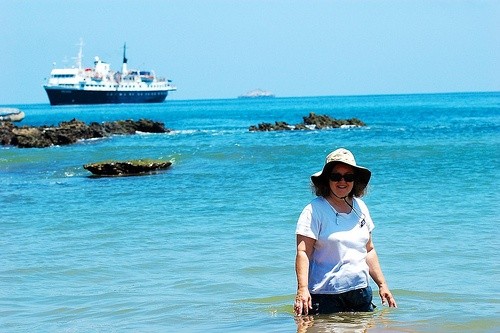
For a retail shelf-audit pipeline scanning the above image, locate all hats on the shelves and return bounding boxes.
[306,145,372,197]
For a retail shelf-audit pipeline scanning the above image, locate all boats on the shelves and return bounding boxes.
[43,37,177,106]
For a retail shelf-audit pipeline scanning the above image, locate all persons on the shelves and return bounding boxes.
[294,148,398,316]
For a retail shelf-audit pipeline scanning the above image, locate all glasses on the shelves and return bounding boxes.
[327,171,360,185]
